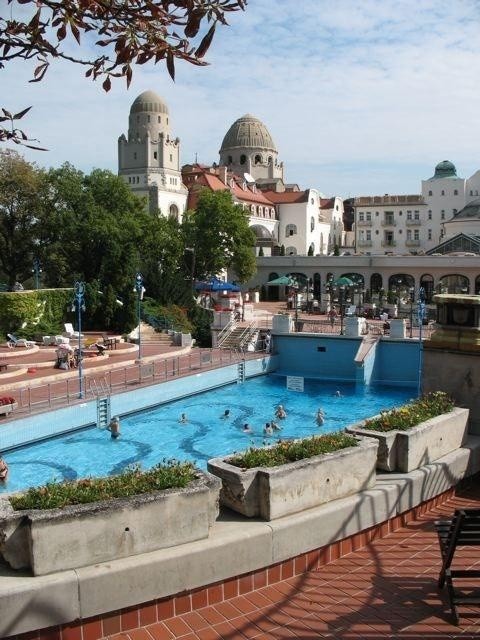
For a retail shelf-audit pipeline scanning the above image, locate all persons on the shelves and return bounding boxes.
[333,390,342,398]
[327,305,338,328]
[220,404,325,447]
[106,415,121,440]
[178,413,188,424]
[0,455,8,484]
[264,330,272,354]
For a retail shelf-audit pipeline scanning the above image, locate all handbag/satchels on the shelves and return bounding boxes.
[59,363,68,370]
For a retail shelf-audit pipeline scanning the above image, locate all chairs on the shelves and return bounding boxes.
[301,301,382,320]
[0,323,118,372]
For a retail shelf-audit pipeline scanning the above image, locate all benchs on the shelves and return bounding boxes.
[432,507,480,627]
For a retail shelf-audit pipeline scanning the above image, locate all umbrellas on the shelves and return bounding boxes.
[265,275,298,300]
[194,276,239,293]
[325,277,357,289]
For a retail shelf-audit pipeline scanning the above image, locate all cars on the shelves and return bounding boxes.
[0,281,26,291]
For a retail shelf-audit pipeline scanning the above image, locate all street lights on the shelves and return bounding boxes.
[69,280,87,400]
[30,257,44,290]
[286,273,416,339]
[132,271,147,364]
[184,247,195,291]
